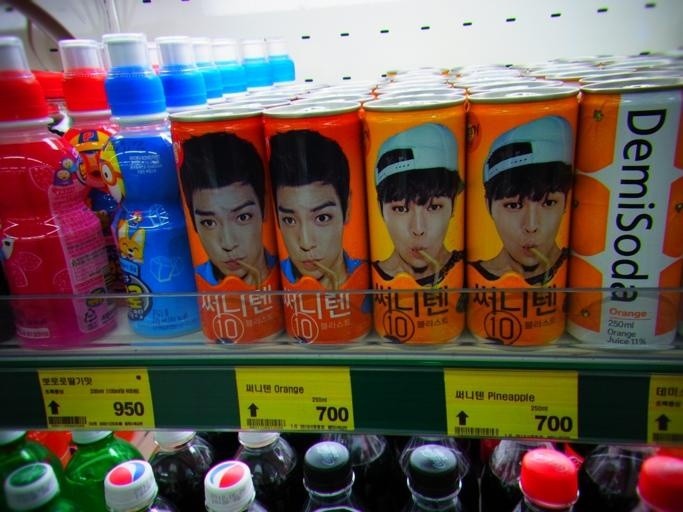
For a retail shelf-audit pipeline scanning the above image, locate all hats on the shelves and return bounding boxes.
[375,124,466,195]
[482,115,575,186]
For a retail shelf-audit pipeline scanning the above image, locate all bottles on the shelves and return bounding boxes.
[0,426,682,511]
[0,31,298,347]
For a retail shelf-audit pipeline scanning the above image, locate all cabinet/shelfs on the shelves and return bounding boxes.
[0,0,683,512]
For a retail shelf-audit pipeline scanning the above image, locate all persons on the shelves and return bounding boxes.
[179,132,278,285]
[370,122,464,285]
[267,129,362,284]
[466,117,574,285]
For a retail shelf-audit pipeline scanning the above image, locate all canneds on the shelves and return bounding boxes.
[168,50,682,349]
